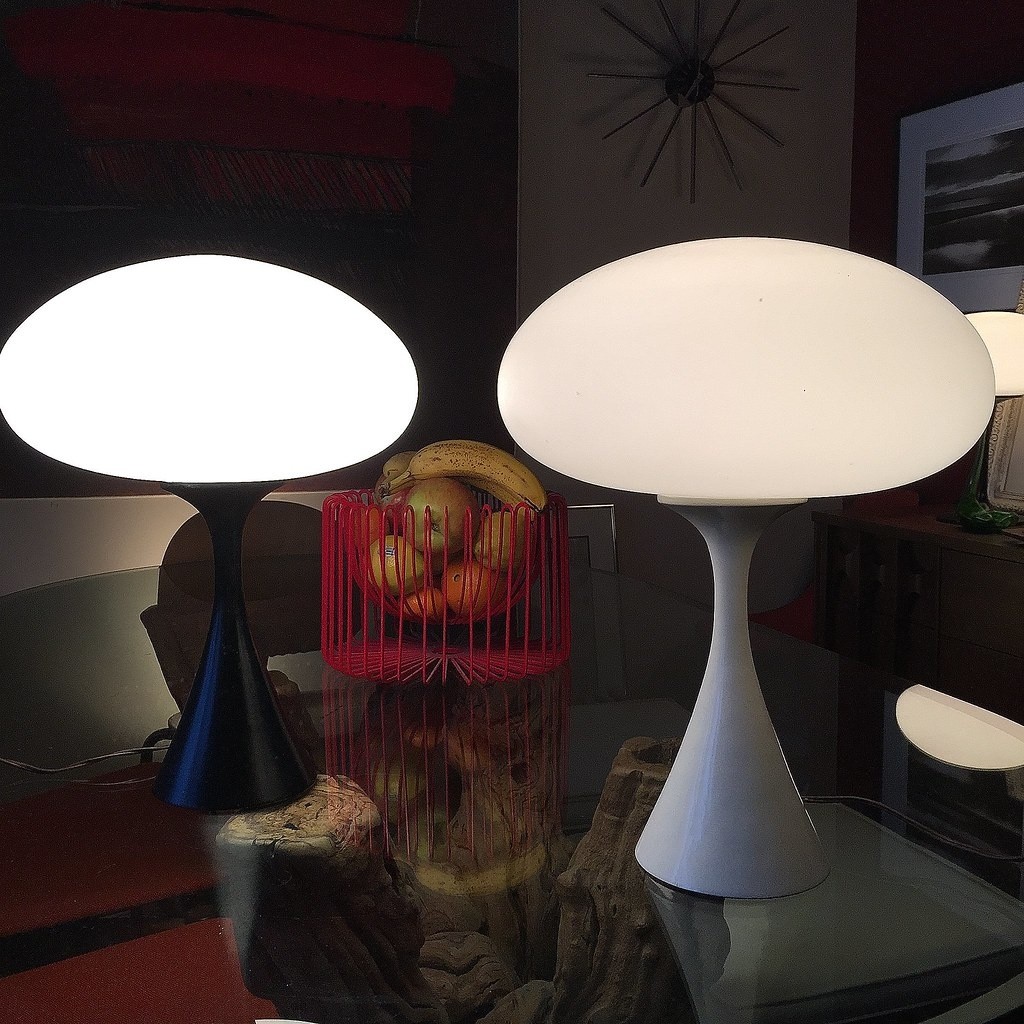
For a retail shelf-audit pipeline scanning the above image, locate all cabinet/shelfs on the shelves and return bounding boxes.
[811,506,1024,724]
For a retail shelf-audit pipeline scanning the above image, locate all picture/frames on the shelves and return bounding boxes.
[897,82,1024,310]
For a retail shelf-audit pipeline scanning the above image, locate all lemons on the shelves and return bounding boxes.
[361,534,427,596]
[471,511,536,572]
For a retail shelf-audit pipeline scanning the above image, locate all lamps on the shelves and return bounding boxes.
[936,310,1024,533]
[0,254,419,819]
[495,236,997,901]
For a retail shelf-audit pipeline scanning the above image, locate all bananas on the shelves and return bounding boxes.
[378,440,547,516]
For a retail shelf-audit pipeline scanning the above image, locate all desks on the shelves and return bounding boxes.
[0,555,1024,1024]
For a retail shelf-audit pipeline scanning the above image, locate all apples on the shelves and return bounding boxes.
[401,478,480,554]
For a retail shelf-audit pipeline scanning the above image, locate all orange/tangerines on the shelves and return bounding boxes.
[346,505,510,621]
